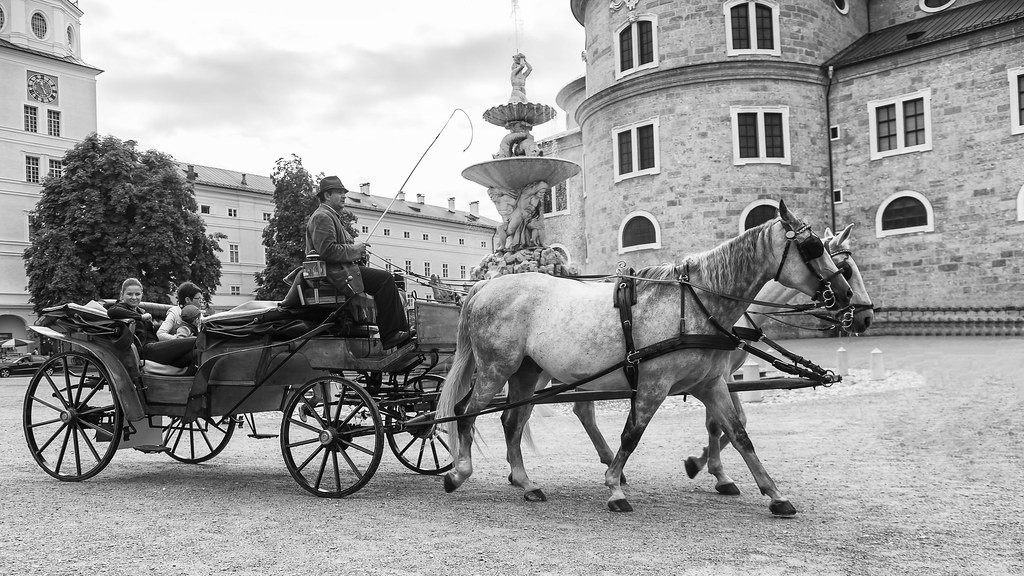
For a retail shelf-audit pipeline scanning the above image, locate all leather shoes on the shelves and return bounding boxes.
[397,329,417,348]
[382,330,412,350]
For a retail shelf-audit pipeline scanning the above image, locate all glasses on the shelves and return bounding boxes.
[189,297,203,302]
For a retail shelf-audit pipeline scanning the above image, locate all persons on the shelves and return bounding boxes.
[22,358,29,363]
[106,278,160,345]
[155,283,205,342]
[32,347,39,355]
[510,52,533,87]
[304,174,416,350]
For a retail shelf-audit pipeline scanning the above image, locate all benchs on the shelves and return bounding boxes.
[96,299,177,336]
[298,266,375,322]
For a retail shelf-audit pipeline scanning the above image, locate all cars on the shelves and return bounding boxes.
[0,355,64,378]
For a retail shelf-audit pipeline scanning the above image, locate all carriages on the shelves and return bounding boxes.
[23,200,876,520]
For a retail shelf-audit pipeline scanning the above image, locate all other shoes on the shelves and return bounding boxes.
[299,407,307,422]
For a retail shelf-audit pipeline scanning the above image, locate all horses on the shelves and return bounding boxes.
[433,198,875,519]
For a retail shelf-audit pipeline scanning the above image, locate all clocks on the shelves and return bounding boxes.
[26,73,58,104]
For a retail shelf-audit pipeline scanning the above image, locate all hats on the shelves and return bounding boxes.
[179,305,201,321]
[317,175,349,195]
[176,281,202,305]
